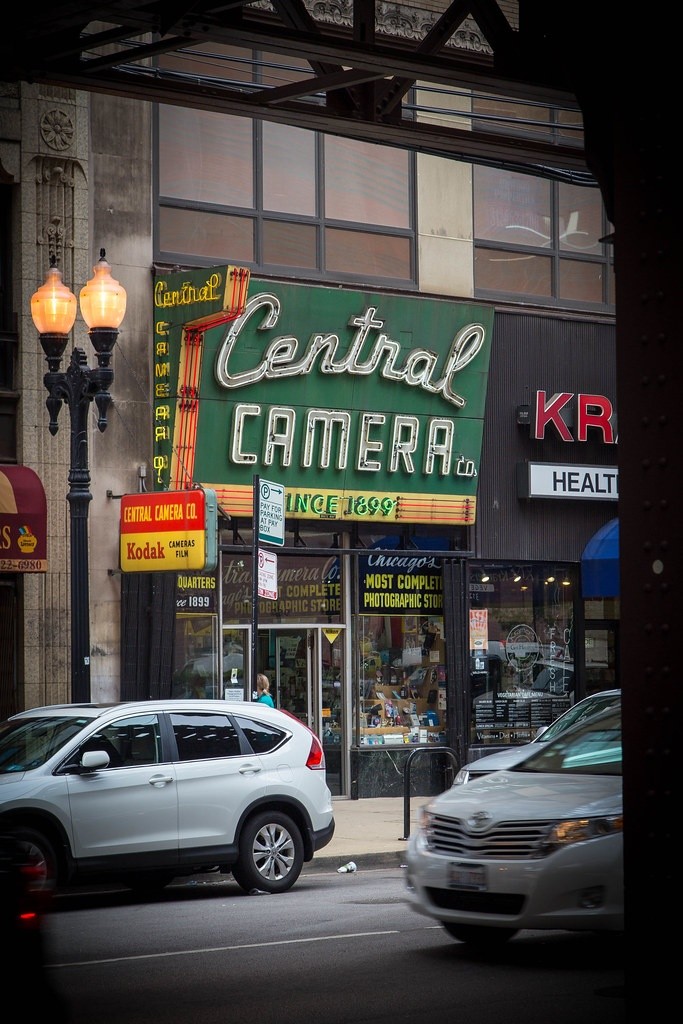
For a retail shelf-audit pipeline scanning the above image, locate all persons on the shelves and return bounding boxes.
[257,673,275,708]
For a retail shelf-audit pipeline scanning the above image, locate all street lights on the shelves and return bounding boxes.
[32,245,128,710]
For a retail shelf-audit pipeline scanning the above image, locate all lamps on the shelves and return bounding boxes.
[480,568,489,582]
[511,568,521,582]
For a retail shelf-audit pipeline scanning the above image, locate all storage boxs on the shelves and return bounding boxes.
[363,713,441,746]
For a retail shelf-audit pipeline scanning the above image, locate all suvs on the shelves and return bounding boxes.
[448,688,622,792]
[406,703,624,946]
[0,696,338,915]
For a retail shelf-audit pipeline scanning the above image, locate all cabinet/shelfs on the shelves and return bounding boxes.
[325,635,444,748]
